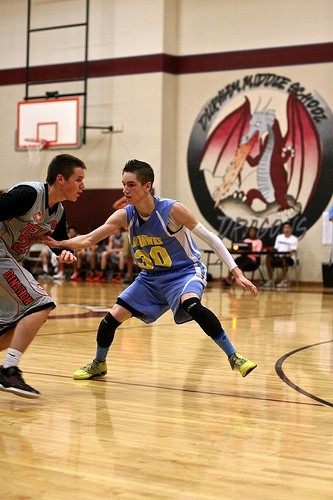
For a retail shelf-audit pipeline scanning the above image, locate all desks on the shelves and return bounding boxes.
[204,249,291,292]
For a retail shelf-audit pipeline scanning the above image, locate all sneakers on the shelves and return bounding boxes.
[227,351,258,377]
[0,365,40,398]
[73,359,107,380]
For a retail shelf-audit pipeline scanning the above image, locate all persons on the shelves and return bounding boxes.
[0,154,86,398]
[41,159,258,379]
[264,222,299,286]
[38,226,134,284]
[224,227,263,286]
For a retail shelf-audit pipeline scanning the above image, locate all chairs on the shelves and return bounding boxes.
[233,255,300,288]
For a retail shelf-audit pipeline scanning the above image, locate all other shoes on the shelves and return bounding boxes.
[221,278,233,286]
[37,270,134,283]
[264,280,272,286]
[276,280,291,287]
